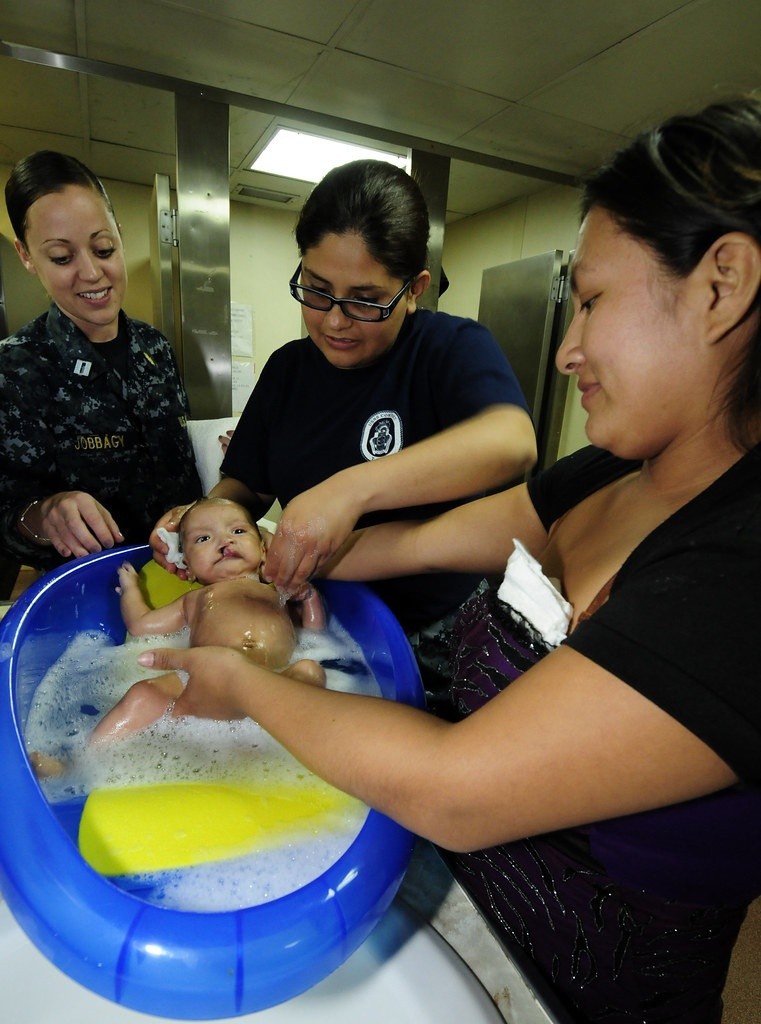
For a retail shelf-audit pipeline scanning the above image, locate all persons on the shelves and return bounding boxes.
[29,497,326,781]
[138,92,761,1024]
[149,159,537,639]
[0,150,234,566]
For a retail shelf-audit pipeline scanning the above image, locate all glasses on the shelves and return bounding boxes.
[289,259,432,322]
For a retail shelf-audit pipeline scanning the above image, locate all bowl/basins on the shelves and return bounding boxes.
[0,545,426,1021]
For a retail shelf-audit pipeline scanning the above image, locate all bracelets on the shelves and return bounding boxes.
[21,500,50,541]
